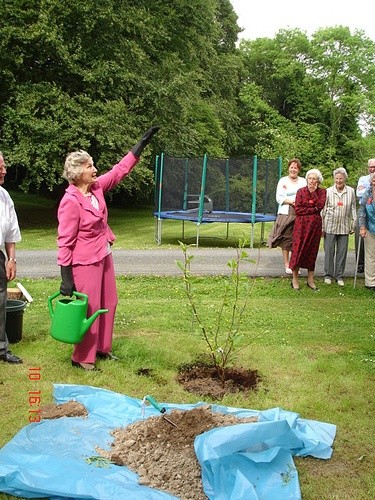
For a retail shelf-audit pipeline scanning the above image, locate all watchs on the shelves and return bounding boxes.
[8,257,16,264]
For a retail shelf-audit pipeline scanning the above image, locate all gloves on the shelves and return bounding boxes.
[60,265,77,297]
[130,126,161,158]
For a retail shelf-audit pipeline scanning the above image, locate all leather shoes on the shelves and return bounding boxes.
[0,351,23,364]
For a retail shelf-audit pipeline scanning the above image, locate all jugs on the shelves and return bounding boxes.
[48,290,109,345]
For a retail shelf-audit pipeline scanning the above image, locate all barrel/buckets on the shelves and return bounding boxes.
[6,299,25,343]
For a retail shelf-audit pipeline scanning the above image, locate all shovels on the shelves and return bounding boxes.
[140,394,179,430]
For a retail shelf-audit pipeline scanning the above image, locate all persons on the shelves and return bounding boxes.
[321,167,357,286]
[267,158,307,274]
[355,158,375,273]
[288,169,327,290]
[358,173,375,291]
[0,150,24,364]
[58,127,161,372]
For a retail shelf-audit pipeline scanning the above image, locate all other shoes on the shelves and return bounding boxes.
[324,278,331,284]
[357,267,364,273]
[71,360,103,373]
[285,268,293,274]
[290,281,301,291]
[307,281,319,291]
[96,352,120,362]
[337,280,345,286]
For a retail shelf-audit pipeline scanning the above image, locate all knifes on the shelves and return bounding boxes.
[145,395,180,428]
